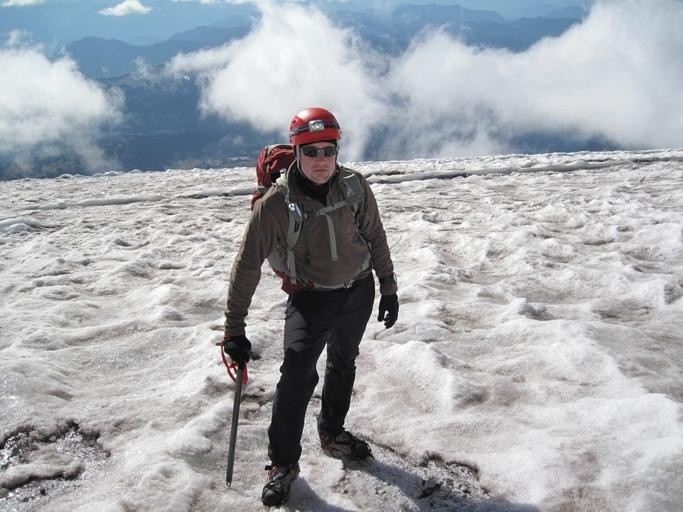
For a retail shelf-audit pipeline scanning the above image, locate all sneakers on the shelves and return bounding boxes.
[261,462,300,507]
[318,428,372,457]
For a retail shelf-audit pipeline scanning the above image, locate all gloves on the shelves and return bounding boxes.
[224,335,251,362]
[377,295,399,329]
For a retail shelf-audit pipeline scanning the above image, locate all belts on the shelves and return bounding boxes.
[307,252,372,289]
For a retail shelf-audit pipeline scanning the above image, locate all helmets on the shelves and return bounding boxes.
[288,107,342,145]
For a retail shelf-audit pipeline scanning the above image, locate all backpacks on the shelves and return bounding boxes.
[251,144,295,213]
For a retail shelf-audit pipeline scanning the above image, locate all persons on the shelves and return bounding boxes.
[224,108,399,506]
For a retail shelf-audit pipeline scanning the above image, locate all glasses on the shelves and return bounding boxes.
[300,146,337,158]
